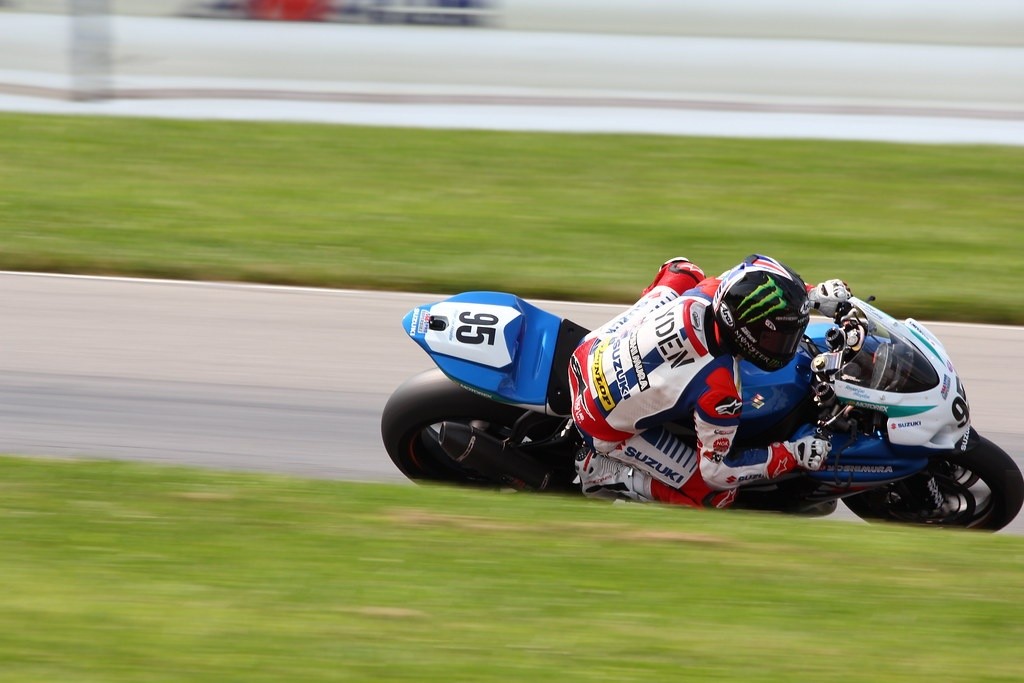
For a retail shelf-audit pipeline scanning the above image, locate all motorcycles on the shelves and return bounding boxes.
[381,290,1023,534]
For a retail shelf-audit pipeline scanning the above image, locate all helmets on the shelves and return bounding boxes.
[711,251,812,371]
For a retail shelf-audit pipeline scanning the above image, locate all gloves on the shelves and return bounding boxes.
[807,279,852,319]
[784,435,831,472]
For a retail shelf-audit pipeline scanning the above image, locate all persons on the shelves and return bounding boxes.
[568,254,853,509]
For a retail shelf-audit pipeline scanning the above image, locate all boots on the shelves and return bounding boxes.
[574,449,654,503]
